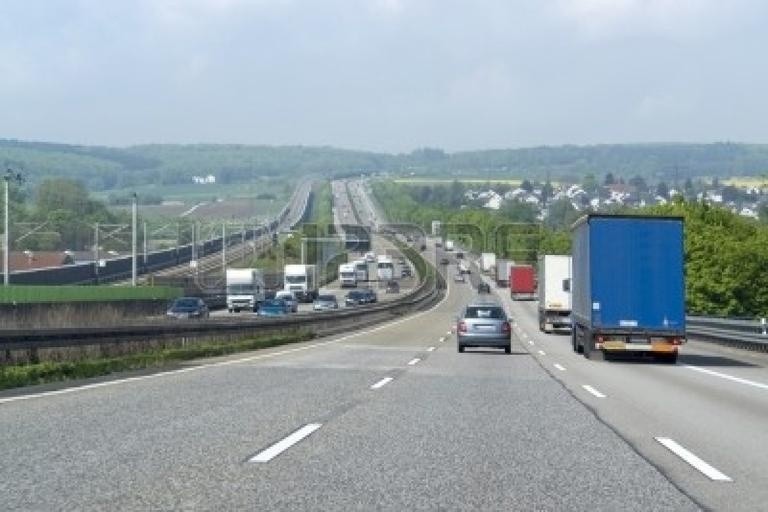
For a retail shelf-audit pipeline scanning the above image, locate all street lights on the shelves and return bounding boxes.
[5,169,22,287]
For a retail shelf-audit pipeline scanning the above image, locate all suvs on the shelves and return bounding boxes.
[456,304,512,355]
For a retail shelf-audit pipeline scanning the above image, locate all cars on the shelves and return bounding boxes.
[385,282,399,293]
[435,237,490,294]
[397,256,411,278]
[167,296,212,321]
[255,290,377,319]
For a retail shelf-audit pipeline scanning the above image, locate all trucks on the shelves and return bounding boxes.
[569,213,687,363]
[337,251,395,287]
[225,267,266,314]
[538,254,572,336]
[478,252,539,302]
[283,264,319,301]
